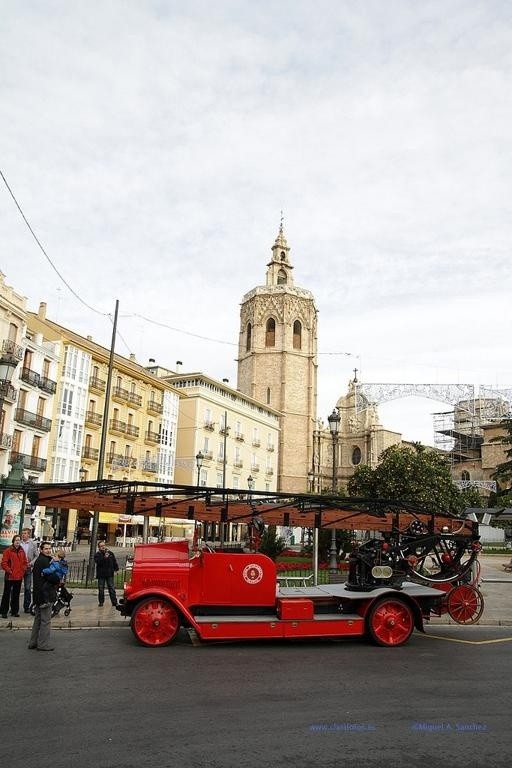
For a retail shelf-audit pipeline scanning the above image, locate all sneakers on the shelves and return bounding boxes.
[25,608,31,613]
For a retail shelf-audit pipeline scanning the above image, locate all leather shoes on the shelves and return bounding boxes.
[98,602,103,606]
[37,645,54,651]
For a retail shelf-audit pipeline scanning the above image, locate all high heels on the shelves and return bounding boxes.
[11,612,20,617]
[2,613,8,618]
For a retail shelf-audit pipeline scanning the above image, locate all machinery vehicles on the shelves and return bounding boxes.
[27,480,485,646]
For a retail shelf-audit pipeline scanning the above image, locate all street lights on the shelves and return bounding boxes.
[247,476,253,502]
[329,409,341,573]
[196,452,204,500]
[307,470,314,494]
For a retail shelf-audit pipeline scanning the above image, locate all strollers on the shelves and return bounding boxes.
[30,582,72,618]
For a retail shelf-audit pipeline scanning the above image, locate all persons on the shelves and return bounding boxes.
[19,528,40,614]
[41,549,67,609]
[2,536,27,617]
[28,542,60,651]
[93,541,120,607]
